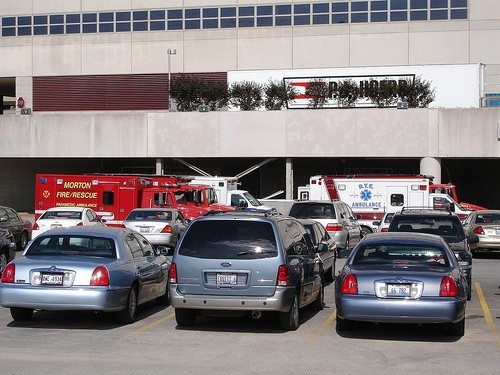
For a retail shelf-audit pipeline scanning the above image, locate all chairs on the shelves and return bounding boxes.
[368,251,390,259]
[492,215,500,221]
[476,218,484,223]
[399,224,413,231]
[438,225,453,234]
[159,214,167,219]
[135,215,144,220]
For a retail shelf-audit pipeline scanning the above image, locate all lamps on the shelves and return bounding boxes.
[21,107,32,114]
[199,104,210,112]
[397,101,408,109]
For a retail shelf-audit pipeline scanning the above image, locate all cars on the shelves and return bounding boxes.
[30,207,112,250]
[0,225,172,325]
[462,210,500,259]
[334,230,470,337]
[0,205,32,279]
[372,211,402,233]
[120,208,192,256]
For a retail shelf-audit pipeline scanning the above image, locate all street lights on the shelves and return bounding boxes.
[165,48,177,112]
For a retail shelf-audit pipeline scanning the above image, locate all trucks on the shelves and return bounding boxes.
[35,172,279,224]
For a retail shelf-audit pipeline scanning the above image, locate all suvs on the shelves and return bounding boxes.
[385,206,479,266]
[287,199,362,257]
[167,207,329,332]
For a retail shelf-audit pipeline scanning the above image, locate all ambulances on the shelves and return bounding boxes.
[297,174,494,239]
